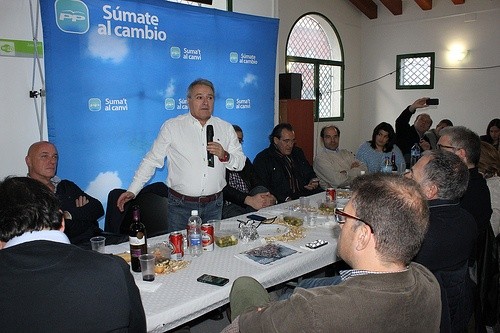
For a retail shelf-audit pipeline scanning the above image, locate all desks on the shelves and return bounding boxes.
[104,188,341,333]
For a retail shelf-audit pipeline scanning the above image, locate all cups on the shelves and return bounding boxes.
[336,199,349,208]
[306,209,318,225]
[207,220,220,236]
[90,236,106,254]
[138,253,156,282]
[300,197,310,213]
[240,225,256,242]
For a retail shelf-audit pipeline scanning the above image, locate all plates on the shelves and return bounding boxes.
[281,212,330,226]
[256,224,290,237]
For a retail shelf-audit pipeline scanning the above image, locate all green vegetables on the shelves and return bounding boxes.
[283,216,304,226]
[318,203,334,212]
[215,235,238,247]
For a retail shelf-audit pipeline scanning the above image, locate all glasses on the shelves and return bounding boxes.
[334,208,374,234]
[436,144,456,150]
[237,219,255,229]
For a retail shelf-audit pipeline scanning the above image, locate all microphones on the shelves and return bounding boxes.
[206,125,214,167]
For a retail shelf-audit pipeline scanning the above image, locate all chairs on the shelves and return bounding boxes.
[101,193,168,244]
[480,206,500,333]
[431,258,482,333]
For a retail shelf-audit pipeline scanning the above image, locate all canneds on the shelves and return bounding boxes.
[201,223,214,251]
[169,232,184,257]
[326,188,337,202]
[187,222,200,248]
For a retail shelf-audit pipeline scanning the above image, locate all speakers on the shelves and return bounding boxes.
[279,73,302,100]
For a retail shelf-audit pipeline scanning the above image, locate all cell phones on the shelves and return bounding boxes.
[247,214,266,221]
[305,240,328,249]
[197,274,229,286]
[426,98,439,105]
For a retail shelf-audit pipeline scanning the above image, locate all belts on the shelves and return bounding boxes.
[169,188,221,202]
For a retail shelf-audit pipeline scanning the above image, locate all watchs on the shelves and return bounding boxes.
[219,150,230,162]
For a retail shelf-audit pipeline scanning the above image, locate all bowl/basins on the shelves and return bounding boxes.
[336,189,353,203]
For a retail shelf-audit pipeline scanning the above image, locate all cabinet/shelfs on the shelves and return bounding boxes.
[279,99,314,164]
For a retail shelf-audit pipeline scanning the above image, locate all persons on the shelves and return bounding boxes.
[25,141,128,250]
[117,78,247,333]
[222,98,500,333]
[0,175,147,333]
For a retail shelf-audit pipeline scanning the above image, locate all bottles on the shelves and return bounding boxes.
[380,156,392,173]
[188,210,203,258]
[390,154,398,174]
[410,143,421,168]
[129,205,147,273]
